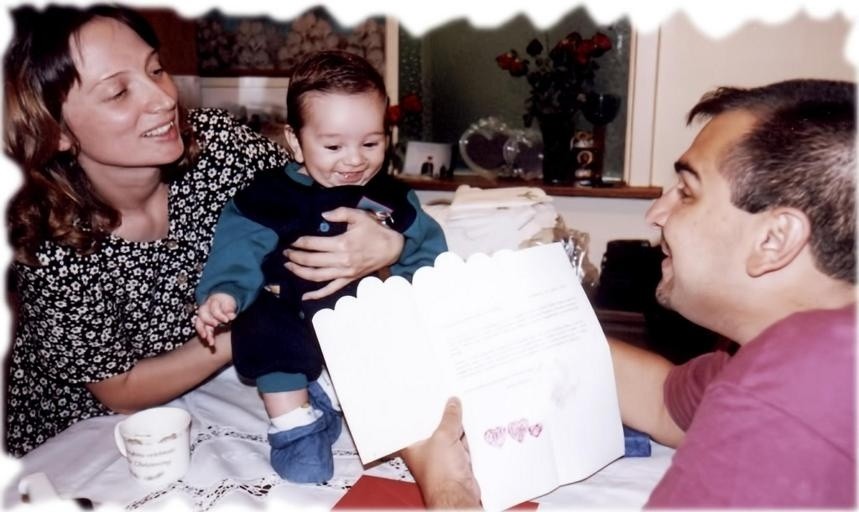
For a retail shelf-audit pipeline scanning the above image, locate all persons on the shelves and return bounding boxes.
[193,48,449,485]
[396,78,854,510]
[1,7,293,458]
[420,155,434,178]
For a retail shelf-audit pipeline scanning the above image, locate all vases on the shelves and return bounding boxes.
[535,109,581,184]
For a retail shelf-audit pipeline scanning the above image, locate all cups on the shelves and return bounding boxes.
[114,408,191,483]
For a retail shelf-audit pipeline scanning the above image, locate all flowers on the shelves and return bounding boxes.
[497,30,615,127]
